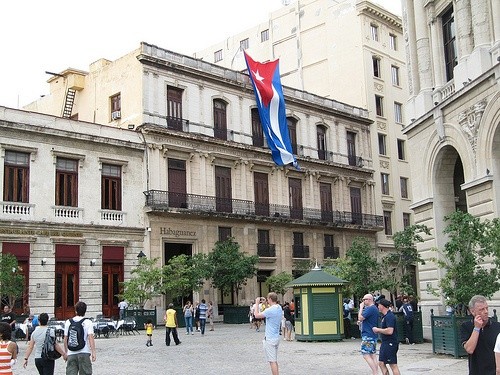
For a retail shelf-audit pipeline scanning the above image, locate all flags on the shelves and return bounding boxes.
[242,48,300,171]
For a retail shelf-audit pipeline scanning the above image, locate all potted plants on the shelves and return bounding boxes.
[431,210,500,359]
[346,224,434,346]
[113,257,163,329]
[207,237,259,325]
[161,253,210,327]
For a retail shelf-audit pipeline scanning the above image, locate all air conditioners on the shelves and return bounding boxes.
[112,112,121,120]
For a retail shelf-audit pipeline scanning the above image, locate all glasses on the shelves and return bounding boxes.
[362,299,371,301]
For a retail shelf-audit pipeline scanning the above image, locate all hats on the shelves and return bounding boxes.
[376,300,391,308]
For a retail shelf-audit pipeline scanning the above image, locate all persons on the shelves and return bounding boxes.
[118,299,128,319]
[0,322,18,375]
[21,313,68,375]
[460,295,500,375]
[342,295,415,346]
[144,320,155,347]
[372,298,401,375]
[63,302,97,375]
[183,299,214,336]
[255,293,282,375]
[251,298,295,341]
[358,294,378,375]
[164,303,181,346]
[0,306,34,339]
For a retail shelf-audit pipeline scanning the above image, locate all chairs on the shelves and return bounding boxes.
[16,316,142,341]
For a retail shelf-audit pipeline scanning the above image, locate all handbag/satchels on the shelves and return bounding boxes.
[41,326,61,360]
[343,304,349,317]
[195,308,200,321]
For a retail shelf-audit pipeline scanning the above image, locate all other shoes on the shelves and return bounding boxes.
[191,331,195,335]
[185,331,189,335]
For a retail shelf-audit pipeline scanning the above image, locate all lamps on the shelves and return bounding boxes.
[137,251,146,265]
[128,124,135,129]
[41,257,46,265]
[90,259,96,266]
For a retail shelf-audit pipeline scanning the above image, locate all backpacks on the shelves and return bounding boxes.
[67,317,90,351]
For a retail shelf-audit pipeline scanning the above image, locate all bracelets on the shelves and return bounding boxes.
[25,358,27,360]
